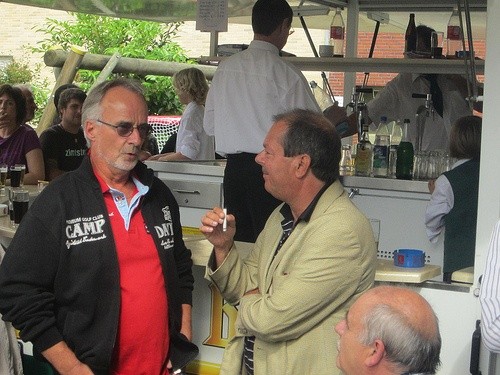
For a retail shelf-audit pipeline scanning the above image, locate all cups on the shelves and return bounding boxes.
[340,145,458,181]
[430,31,444,59]
[1,162,51,231]
[319,45,334,57]
[367,218,381,252]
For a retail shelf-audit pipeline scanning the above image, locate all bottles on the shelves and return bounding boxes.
[345,85,369,140]
[371,117,390,178]
[353,124,373,177]
[404,14,417,57]
[446,5,463,59]
[329,8,344,58]
[396,118,414,180]
[309,80,349,136]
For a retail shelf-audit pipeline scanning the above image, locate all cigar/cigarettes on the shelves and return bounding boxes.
[223,207,229,232]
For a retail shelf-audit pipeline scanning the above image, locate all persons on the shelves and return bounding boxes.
[144,67,218,161]
[199,107,377,375]
[335,23,482,154]
[202,0,348,242]
[424,115,482,283]
[332,286,442,375]
[0,78,197,374]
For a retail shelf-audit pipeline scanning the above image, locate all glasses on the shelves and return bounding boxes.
[96,119,152,138]
